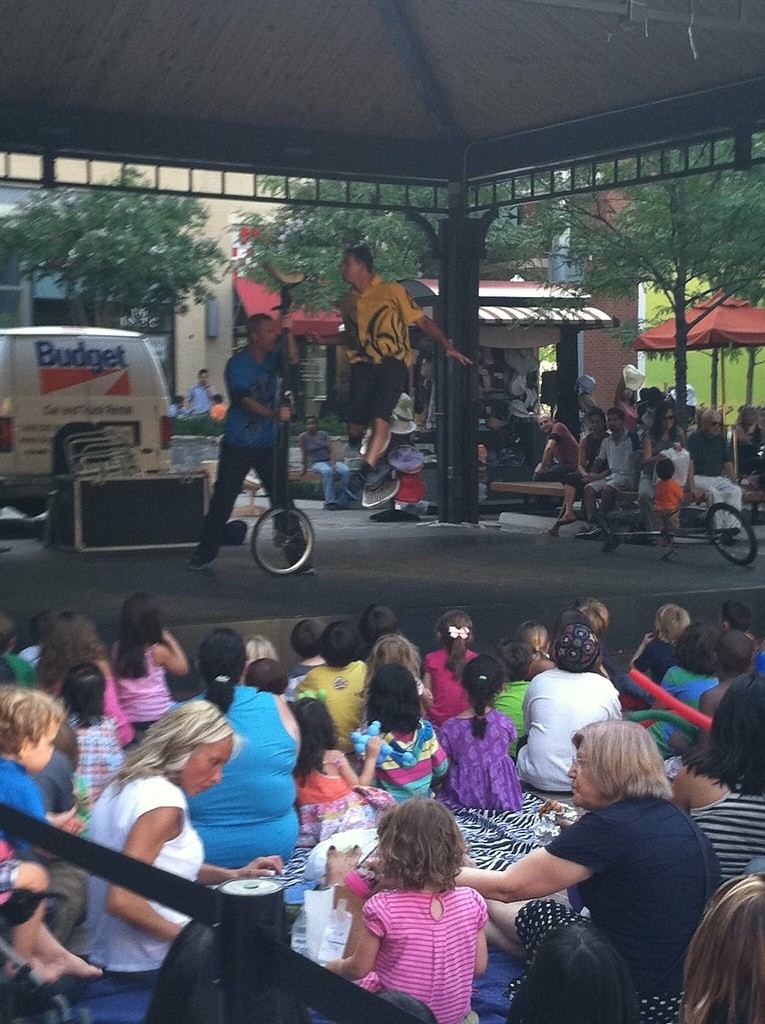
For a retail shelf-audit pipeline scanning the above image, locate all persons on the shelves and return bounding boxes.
[505,926,642,1024]
[186,313,315,574]
[188,368,214,415]
[0,592,765,1024]
[210,394,228,420]
[678,873,765,1024]
[168,395,188,418]
[304,246,474,500]
[359,364,765,561]
[298,416,349,510]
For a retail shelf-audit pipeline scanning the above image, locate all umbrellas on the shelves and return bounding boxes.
[634,290,765,403]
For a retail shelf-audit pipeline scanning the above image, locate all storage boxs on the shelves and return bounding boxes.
[53,472,210,553]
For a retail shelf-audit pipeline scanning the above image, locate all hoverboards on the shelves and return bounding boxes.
[250,373,316,577]
[602,500,759,567]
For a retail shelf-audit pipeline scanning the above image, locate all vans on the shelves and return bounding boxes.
[0,327,174,516]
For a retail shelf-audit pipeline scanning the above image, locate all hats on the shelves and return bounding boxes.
[362,391,424,510]
[555,623,599,672]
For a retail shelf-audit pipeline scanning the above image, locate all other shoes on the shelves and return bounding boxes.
[350,435,361,453]
[348,463,375,492]
[188,552,219,572]
[721,536,739,546]
[575,523,602,540]
[326,502,336,510]
[661,549,677,561]
[301,564,313,574]
[719,528,740,540]
[602,536,622,552]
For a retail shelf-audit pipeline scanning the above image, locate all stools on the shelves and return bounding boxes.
[232,475,269,518]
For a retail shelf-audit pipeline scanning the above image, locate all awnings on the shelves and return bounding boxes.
[478,305,620,329]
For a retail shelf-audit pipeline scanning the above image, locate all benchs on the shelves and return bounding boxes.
[489,480,765,526]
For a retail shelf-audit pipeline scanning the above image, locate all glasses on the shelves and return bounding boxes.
[662,415,674,421]
[706,421,723,427]
[572,756,588,767]
